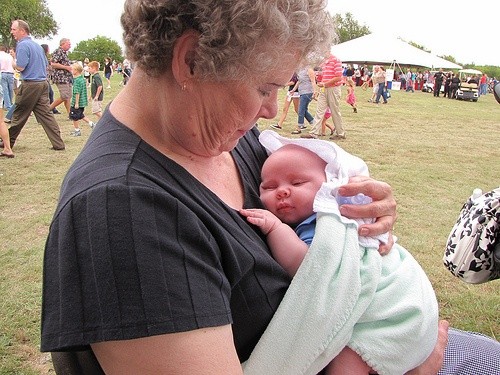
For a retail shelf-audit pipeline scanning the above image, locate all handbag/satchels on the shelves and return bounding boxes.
[361,82,369,91]
[383,90,391,99]
[443,188,500,284]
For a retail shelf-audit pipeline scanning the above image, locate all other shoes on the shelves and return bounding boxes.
[53,110,63,114]
[0,150,15,159]
[0,140,14,149]
[319,133,326,136]
[4,119,12,124]
[373,100,379,104]
[48,145,66,151]
[70,129,81,136]
[270,124,282,130]
[367,98,374,102]
[353,108,358,113]
[300,125,307,130]
[382,101,387,104]
[329,126,336,136]
[107,85,111,88]
[291,131,301,134]
[90,122,95,129]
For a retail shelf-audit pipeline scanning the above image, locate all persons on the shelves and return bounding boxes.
[494,82,500,104]
[9,46,19,94]
[69,63,95,137]
[40,0,500,375]
[88,61,103,118]
[444,71,498,99]
[29,44,61,116]
[0,42,17,110]
[394,68,448,97]
[49,38,73,113]
[83,57,91,86]
[343,63,391,104]
[346,79,358,113]
[0,19,65,150]
[271,44,346,140]
[104,57,133,88]
[240,144,437,375]
[0,63,14,158]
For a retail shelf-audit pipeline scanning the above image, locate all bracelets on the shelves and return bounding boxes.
[322,82,326,88]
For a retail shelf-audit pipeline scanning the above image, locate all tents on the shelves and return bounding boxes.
[331,32,462,70]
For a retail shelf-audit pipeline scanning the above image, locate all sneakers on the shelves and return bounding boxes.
[329,135,346,140]
[301,134,318,139]
